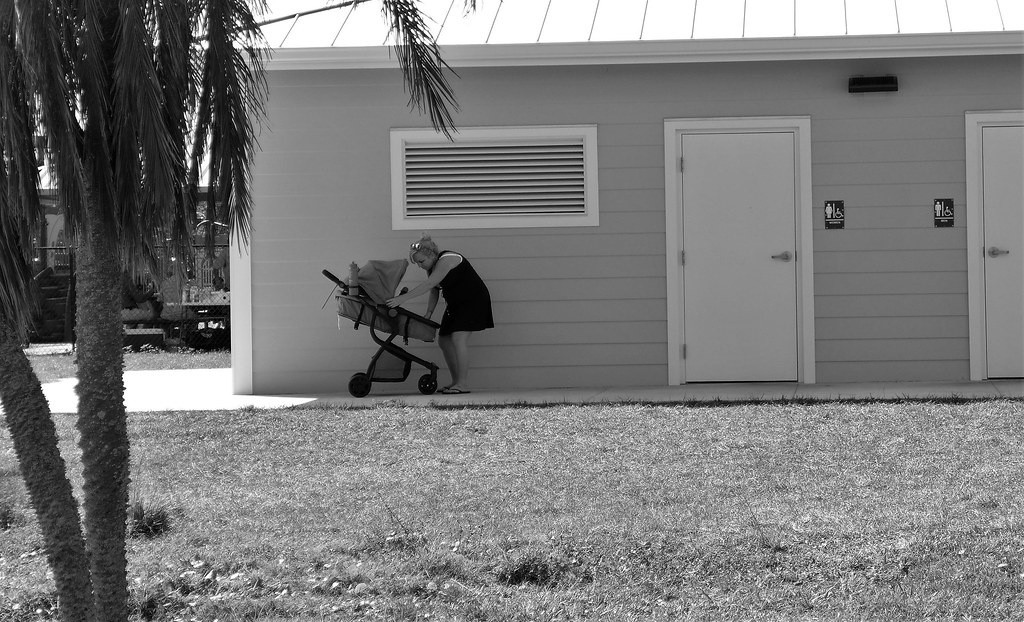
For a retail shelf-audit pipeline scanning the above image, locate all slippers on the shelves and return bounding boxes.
[436,387,471,394]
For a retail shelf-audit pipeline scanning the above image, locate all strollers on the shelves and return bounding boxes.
[322,259,442,398]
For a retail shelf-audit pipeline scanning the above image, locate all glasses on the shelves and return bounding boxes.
[411,243,432,251]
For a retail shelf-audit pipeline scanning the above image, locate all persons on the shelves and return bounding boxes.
[386,236,494,394]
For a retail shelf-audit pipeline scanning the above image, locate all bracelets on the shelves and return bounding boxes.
[427,310,433,313]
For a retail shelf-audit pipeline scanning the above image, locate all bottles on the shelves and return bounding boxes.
[348,261,359,295]
[183,282,190,303]
